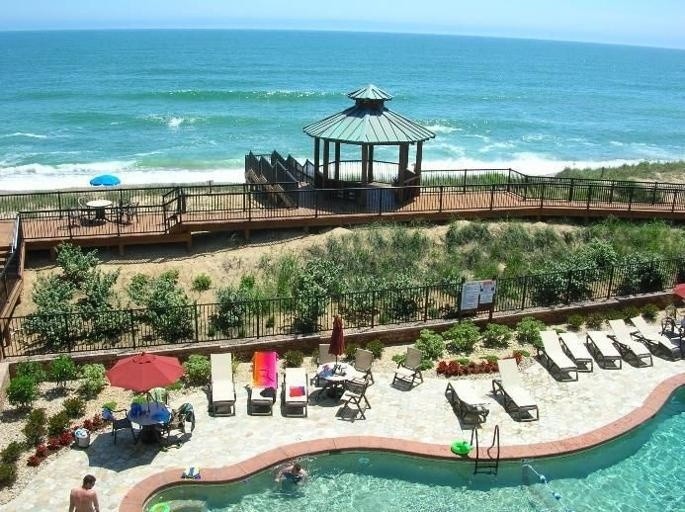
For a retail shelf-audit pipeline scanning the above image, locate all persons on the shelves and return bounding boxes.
[68,474,100,512]
[275,463,307,483]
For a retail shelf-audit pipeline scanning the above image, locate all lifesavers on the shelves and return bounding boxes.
[452,440,472,454]
[150,503,171,512]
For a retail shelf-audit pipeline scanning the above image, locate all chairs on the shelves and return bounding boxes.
[392,348,423,389]
[210,353,236,416]
[539,306,685,384]
[492,358,539,421]
[126,202,140,223]
[104,406,137,444]
[445,381,489,424]
[246,352,278,416]
[163,403,195,436]
[281,368,308,417]
[78,197,89,208]
[311,345,374,419]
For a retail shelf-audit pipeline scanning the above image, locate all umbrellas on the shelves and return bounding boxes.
[89,175,120,186]
[326,315,346,356]
[674,283,685,316]
[105,350,181,409]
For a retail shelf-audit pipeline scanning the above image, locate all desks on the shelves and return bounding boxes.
[128,402,171,426]
[86,200,112,218]
